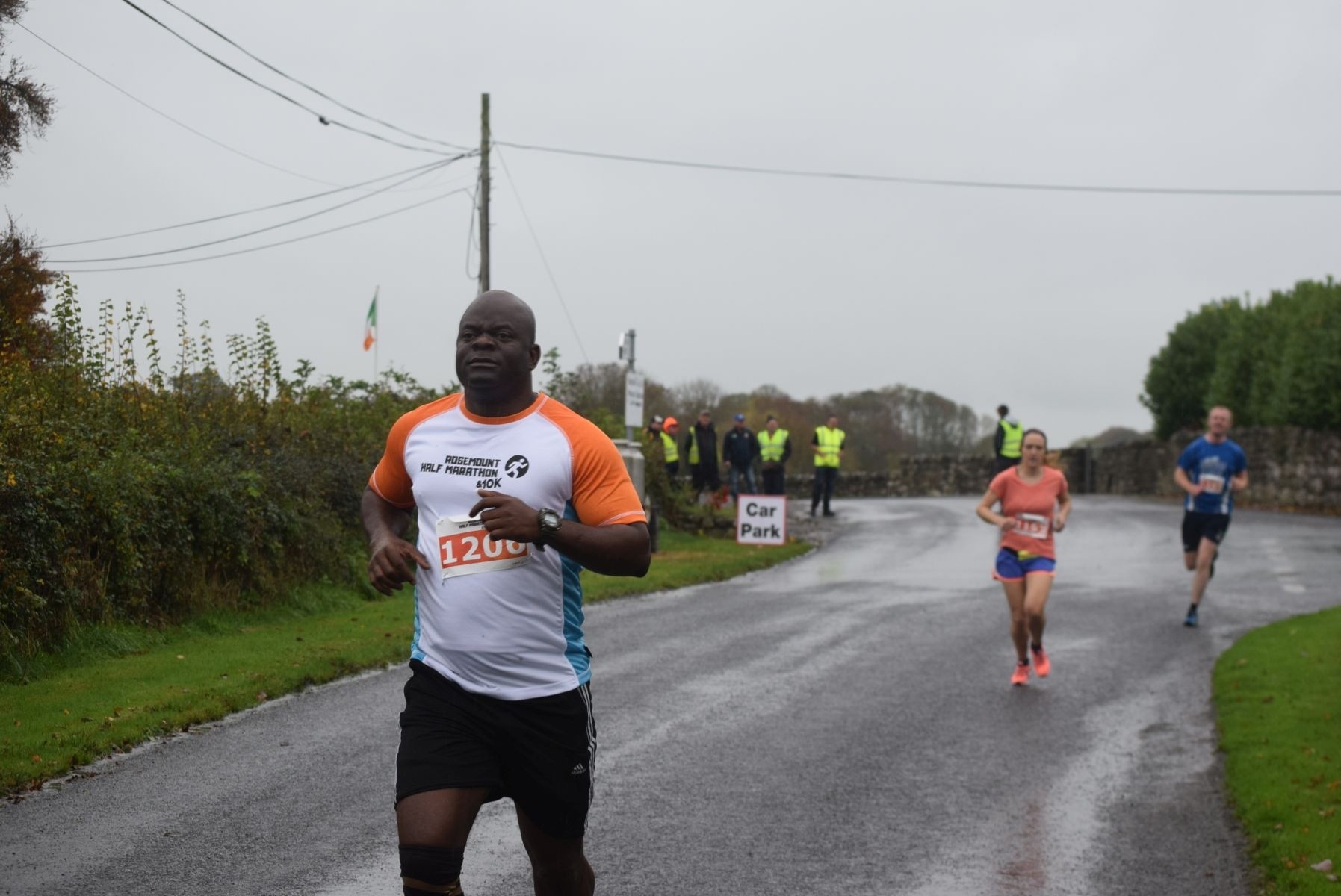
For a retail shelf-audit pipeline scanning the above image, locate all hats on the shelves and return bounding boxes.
[651,416,662,423]
[699,409,711,417]
[767,414,779,423]
[735,414,745,420]
[664,417,680,431]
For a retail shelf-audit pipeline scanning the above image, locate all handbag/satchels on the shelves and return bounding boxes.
[761,461,773,469]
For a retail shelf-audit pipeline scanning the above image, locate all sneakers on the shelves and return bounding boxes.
[1209,550,1218,576]
[1183,610,1196,626]
[1011,664,1031,686]
[1031,645,1050,678]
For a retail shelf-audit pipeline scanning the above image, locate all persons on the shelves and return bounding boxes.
[360,290,651,896]
[977,429,1071,685]
[995,405,1023,472]
[643,410,790,508]
[808,416,846,517]
[1174,405,1249,627]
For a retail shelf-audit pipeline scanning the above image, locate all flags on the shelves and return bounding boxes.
[364,295,384,360]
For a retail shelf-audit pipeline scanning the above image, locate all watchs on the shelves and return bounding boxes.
[533,508,562,552]
